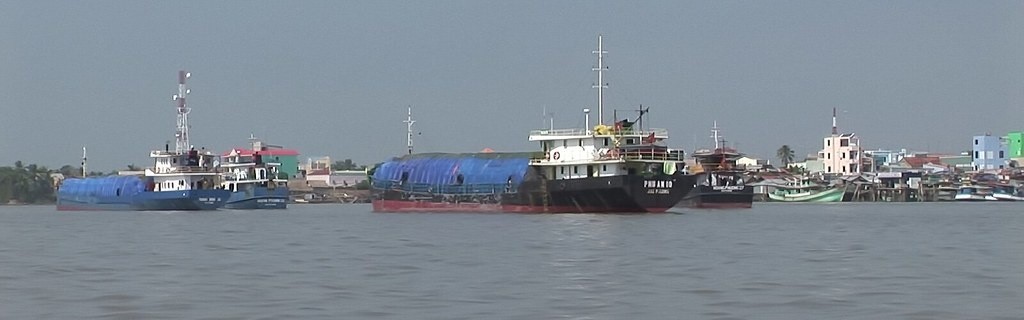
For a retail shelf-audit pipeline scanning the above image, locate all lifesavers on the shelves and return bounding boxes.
[553,151,560,160]
[560,182,566,190]
[610,149,620,160]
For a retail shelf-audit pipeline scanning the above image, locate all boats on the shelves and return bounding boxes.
[671,118,757,209]
[54,70,232,212]
[992,184,1024,201]
[765,183,848,203]
[954,186,999,202]
[365,33,698,215]
[219,132,291,209]
